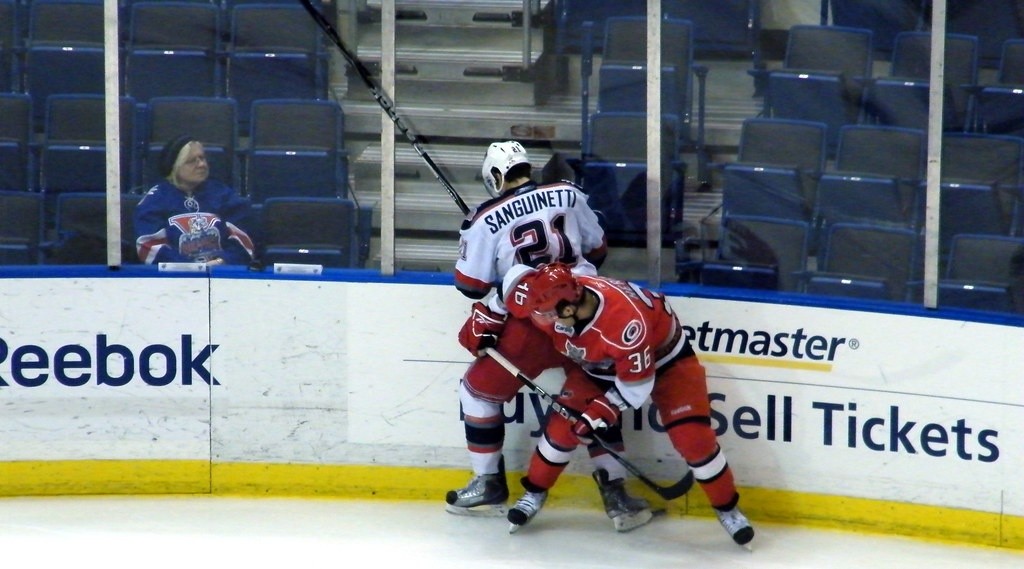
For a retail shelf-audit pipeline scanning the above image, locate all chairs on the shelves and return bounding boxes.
[0,0,371,270]
[544,0,1024,313]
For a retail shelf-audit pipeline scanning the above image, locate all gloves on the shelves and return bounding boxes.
[459,301,507,357]
[570,395,620,444]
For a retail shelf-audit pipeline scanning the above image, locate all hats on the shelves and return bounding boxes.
[158,134,197,177]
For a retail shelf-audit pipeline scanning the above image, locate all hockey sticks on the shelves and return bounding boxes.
[301,0,471,218]
[485,345,696,502]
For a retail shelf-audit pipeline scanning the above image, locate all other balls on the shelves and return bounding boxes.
[652,508,667,517]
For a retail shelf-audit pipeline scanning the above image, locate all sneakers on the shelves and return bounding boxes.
[716,506,755,551]
[445,454,510,516]
[591,468,653,532]
[507,476,548,534]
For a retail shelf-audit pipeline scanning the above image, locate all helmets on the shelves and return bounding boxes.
[506,260,581,315]
[480,140,532,198]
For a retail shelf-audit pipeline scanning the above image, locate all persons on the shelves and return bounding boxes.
[131,134,262,267]
[445,140,652,517]
[458,261,755,544]
